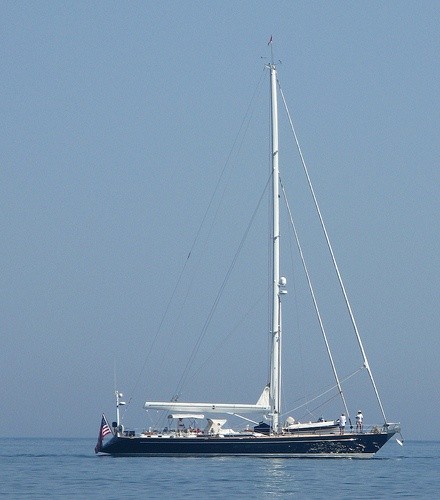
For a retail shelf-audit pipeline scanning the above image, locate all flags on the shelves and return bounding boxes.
[95,414,112,453]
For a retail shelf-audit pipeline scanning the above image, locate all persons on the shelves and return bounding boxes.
[355,410,363,434]
[186,426,201,433]
[338,413,347,434]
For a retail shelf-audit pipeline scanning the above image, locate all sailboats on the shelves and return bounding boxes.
[93,35,402,460]
